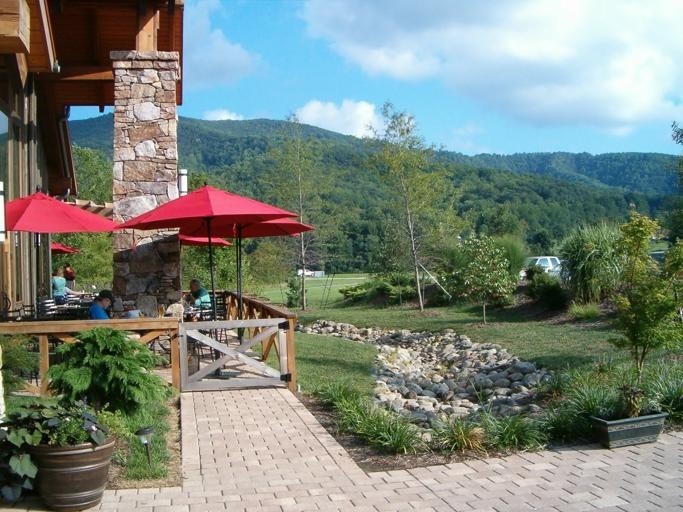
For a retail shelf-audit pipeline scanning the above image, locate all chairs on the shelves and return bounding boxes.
[0,293,229,386]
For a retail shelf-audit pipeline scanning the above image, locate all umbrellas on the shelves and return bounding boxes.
[51,242,80,255]
[114,185,316,376]
[5,191,121,320]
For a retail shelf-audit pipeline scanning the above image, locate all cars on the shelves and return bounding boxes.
[519,256,571,281]
[297,269,314,276]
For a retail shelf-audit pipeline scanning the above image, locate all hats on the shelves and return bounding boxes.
[99,290,112,301]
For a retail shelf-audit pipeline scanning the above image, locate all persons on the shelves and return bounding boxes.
[50,264,81,305]
[88,290,113,319]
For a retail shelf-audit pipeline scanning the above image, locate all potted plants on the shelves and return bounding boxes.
[0,395,115,511]
[587,385,669,448]
